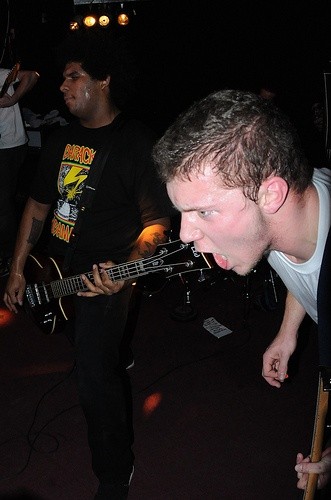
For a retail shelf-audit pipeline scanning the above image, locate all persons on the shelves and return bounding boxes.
[152,89,331,491]
[3,53,172,500]
[0,68,41,277]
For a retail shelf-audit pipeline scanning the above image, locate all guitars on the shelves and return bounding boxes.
[21,228,215,336]
[302,363,330,500]
[0,62,20,98]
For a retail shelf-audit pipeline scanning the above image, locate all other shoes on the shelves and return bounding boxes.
[142,273,168,294]
[93,464,135,500]
